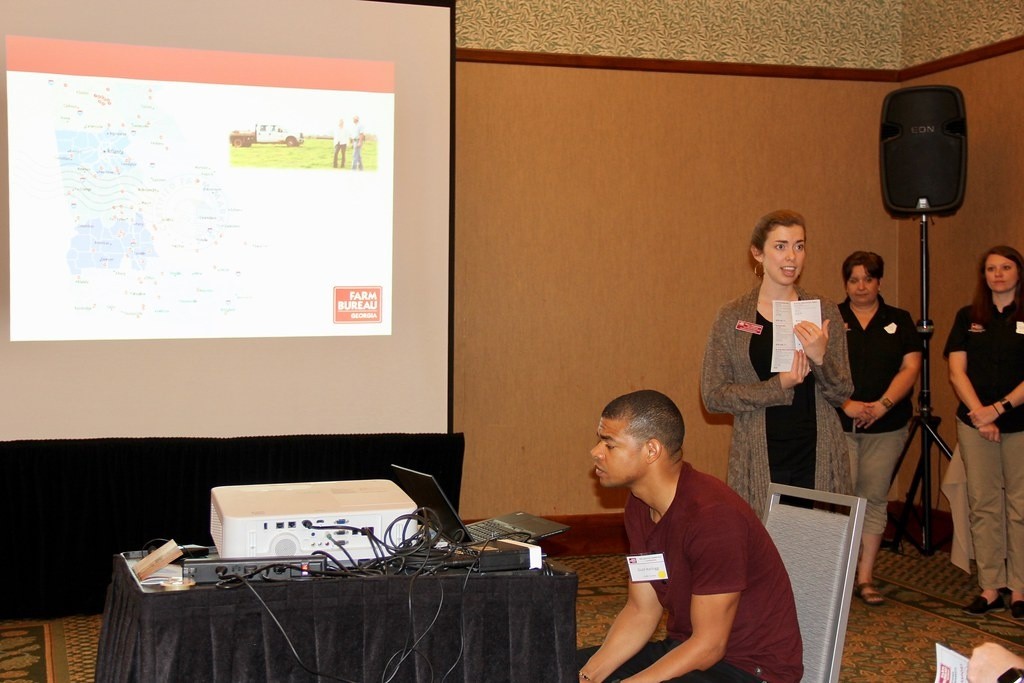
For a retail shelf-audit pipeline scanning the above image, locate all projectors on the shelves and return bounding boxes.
[210,478,418,561]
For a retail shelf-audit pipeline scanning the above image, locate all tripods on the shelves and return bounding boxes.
[887,217,953,556]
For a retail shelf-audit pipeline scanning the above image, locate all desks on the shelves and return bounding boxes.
[95,552,579,683]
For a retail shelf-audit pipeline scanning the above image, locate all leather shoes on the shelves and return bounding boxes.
[961,593,1006,616]
[1009,594,1024,620]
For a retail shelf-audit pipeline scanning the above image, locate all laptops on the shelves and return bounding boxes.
[391,464,572,544]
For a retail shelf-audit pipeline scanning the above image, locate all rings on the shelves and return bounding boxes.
[810,329,813,332]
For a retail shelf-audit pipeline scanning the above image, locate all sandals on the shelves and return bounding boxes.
[854,580,885,606]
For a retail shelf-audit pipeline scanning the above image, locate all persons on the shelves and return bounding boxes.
[943,245,1024,621]
[833,251,923,605]
[332,118,349,168]
[967,642,1024,683]
[700,208,854,522]
[629,558,636,563]
[351,116,364,170]
[577,390,804,683]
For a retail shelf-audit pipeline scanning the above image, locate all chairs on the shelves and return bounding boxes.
[756,482,867,683]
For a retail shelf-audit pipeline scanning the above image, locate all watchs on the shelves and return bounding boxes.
[1000,398,1013,412]
[880,396,894,409]
[997,667,1024,683]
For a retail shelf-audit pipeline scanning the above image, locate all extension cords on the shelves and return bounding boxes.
[182,556,327,584]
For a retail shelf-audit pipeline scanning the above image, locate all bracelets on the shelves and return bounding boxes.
[993,405,1001,416]
[579,672,590,681]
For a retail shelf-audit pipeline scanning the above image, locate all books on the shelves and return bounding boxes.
[934,643,970,683]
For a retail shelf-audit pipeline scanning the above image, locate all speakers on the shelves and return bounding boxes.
[878,84,968,219]
